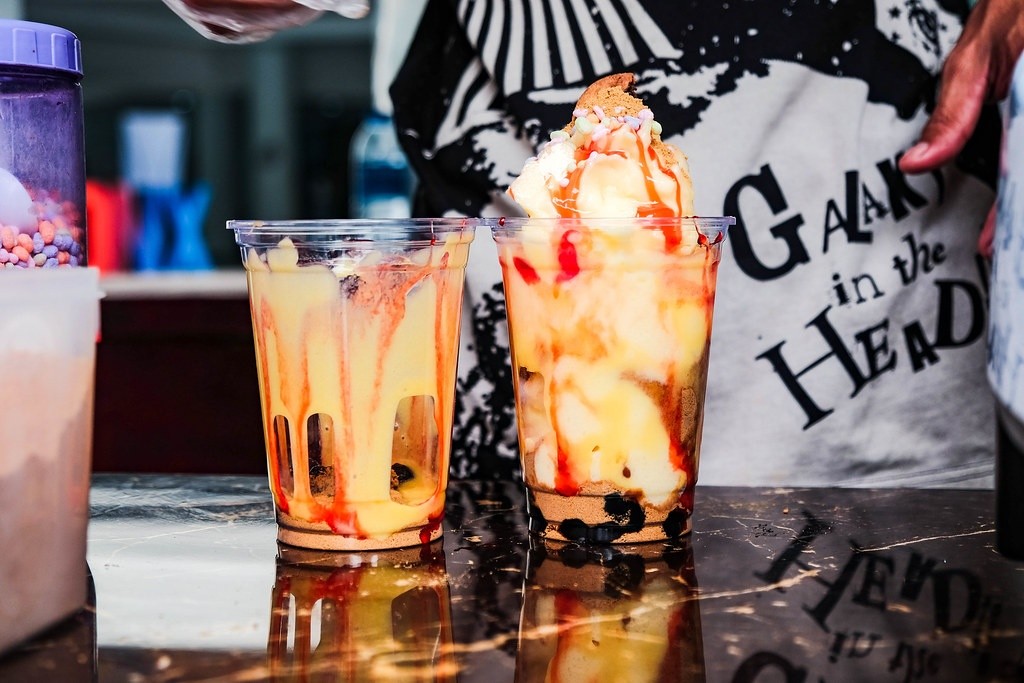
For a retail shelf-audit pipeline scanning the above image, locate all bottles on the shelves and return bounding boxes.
[352,114,409,222]
[0,19,89,270]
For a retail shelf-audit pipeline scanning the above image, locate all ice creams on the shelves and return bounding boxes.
[490,71,719,547]
[242,224,475,552]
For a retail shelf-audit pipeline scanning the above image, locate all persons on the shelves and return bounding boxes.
[166,0,1024,488]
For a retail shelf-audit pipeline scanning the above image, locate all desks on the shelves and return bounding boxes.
[1,477,1024,683]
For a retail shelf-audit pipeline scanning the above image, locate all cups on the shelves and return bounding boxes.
[266,549,457,682]
[486,216,736,543]
[227,221,479,550]
[515,545,707,683]
